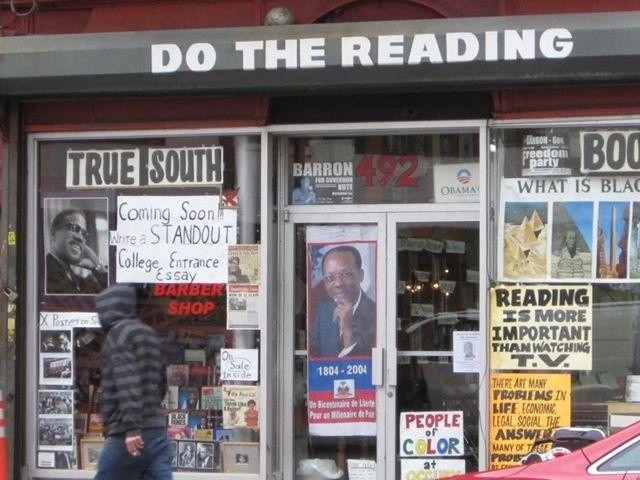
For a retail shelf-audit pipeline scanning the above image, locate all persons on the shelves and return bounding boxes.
[293,177,315,205]
[169,366,186,386]
[179,430,191,440]
[198,447,212,469]
[45,363,71,378]
[244,400,258,427]
[38,393,71,414]
[225,408,241,426]
[558,231,581,261]
[58,333,71,353]
[229,256,252,284]
[221,435,229,442]
[92,283,174,480]
[311,246,376,358]
[48,206,107,295]
[236,454,247,464]
[39,420,72,446]
[464,342,476,358]
[181,390,199,410]
[41,334,56,352]
[180,442,194,469]
[194,416,208,429]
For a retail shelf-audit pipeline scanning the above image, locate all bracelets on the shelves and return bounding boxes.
[92,261,103,275]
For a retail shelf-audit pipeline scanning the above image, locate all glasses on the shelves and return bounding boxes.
[56,223,89,238]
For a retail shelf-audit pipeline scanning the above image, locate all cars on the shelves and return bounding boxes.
[571,382,616,431]
[434,419,640,480]
[296,312,584,416]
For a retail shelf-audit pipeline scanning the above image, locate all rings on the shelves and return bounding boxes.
[132,453,137,456]
